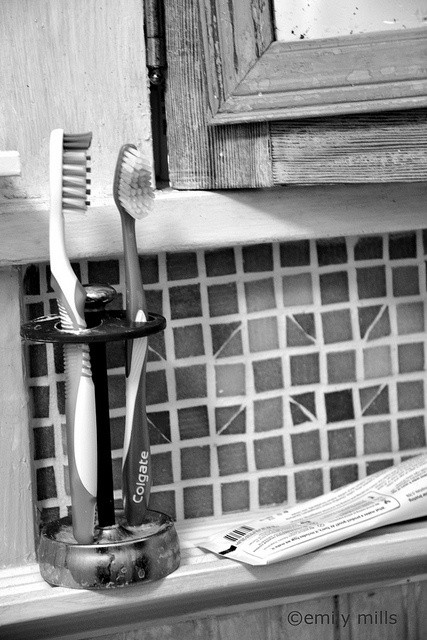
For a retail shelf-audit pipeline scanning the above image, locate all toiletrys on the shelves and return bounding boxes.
[196,454,427,569]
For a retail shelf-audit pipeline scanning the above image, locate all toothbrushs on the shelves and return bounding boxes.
[48,127,98,544]
[112,144,154,526]
